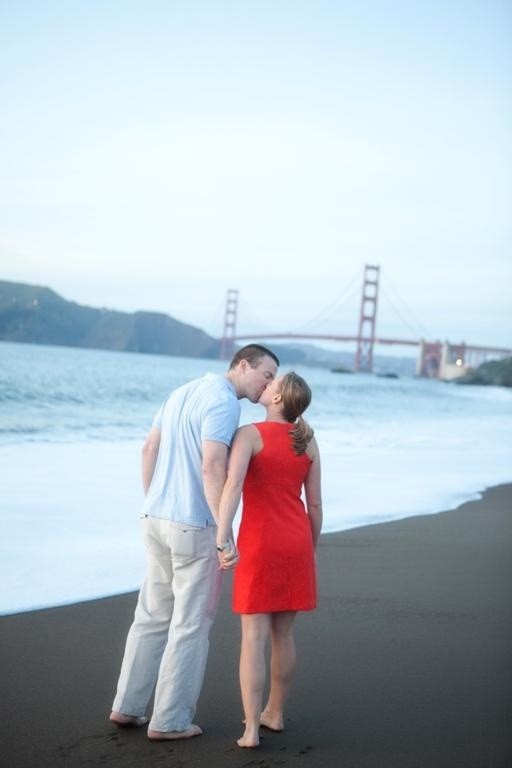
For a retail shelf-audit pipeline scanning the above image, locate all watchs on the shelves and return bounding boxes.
[215,542,231,552]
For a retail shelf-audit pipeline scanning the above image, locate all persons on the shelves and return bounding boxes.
[215,370,324,748]
[106,343,281,741]
